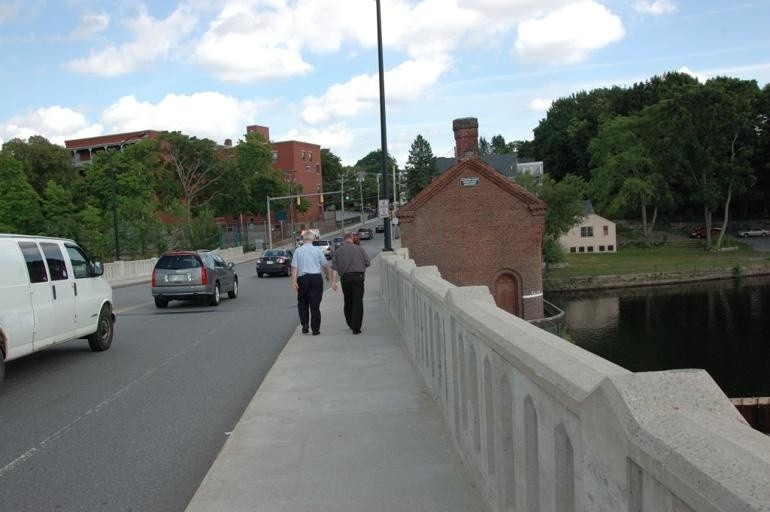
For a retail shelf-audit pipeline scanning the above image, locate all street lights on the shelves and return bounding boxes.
[109,132,148,260]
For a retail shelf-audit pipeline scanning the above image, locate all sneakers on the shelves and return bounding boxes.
[301,327,320,335]
[351,326,361,335]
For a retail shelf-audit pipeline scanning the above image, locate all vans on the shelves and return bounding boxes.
[0,233,115,389]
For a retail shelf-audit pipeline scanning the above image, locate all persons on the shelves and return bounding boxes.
[330,231,371,335]
[289,231,332,335]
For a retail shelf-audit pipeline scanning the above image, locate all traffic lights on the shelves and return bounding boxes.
[343,191,351,200]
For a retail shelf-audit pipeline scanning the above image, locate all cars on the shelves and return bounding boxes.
[737,227,770,237]
[376,223,384,233]
[256,249,292,278]
[358,227,373,240]
[313,232,359,259]
[691,227,721,238]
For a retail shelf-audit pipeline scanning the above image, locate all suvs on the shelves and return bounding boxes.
[152,250,238,306]
